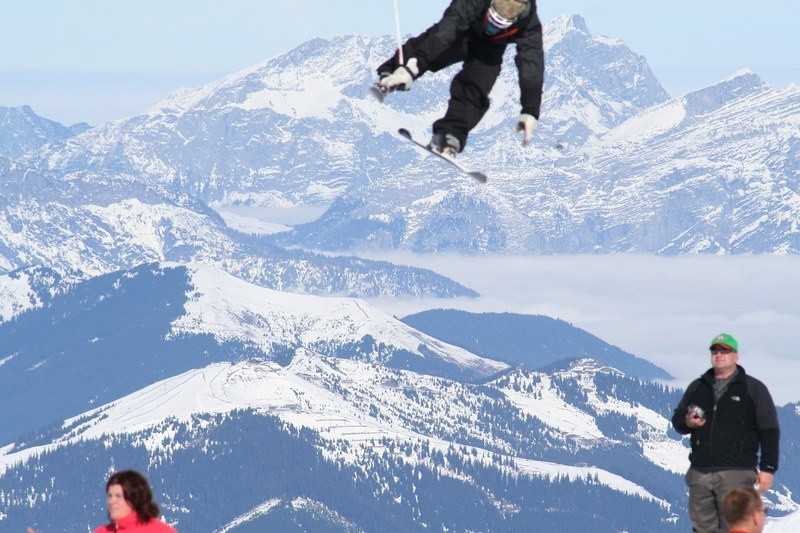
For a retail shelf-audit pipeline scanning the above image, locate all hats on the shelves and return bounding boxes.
[710,334,738,352]
[492,1,527,19]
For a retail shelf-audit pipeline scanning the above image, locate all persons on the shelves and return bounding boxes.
[723,487,765,533]
[673,333,781,533]
[93,471,178,532]
[371,0,545,158]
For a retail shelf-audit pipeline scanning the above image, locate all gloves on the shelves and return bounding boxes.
[516,114,536,144]
[386,58,420,91]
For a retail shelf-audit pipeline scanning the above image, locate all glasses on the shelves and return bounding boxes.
[710,347,731,354]
[487,8,513,28]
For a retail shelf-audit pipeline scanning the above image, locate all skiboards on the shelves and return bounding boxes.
[368,84,488,184]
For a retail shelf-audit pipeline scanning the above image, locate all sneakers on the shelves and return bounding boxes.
[427,134,457,158]
[370,73,394,101]
